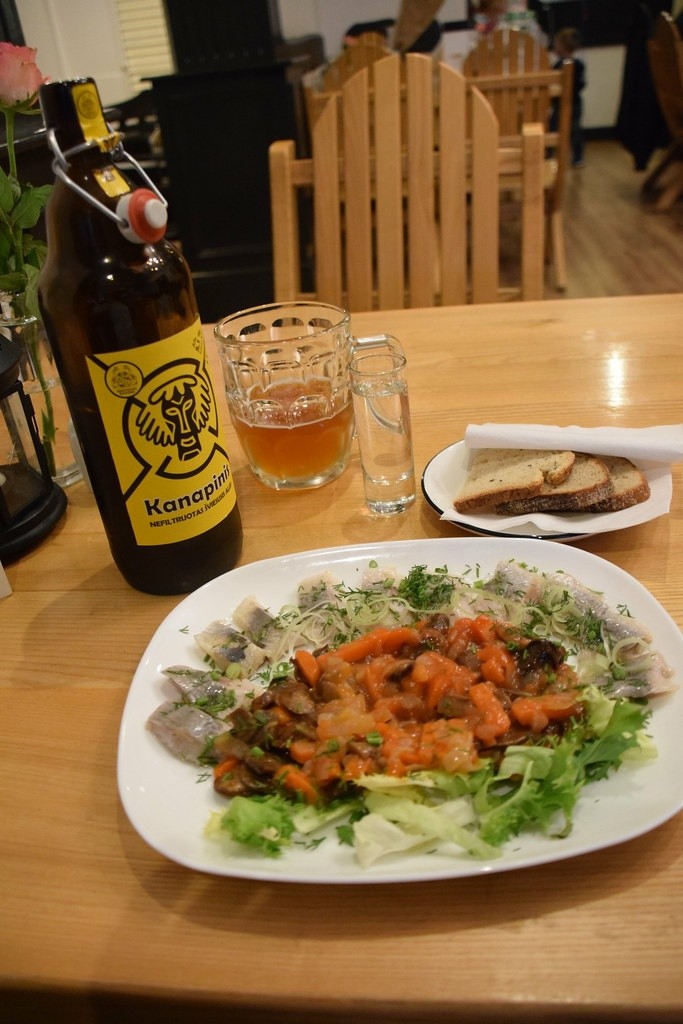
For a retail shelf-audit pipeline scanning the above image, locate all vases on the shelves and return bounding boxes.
[0,289,82,487]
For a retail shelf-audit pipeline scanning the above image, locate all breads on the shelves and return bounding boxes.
[455,446,650,515]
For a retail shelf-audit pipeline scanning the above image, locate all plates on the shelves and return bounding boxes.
[114,538,683,882]
[420,437,596,543]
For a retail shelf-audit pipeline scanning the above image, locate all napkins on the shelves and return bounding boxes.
[438,421,682,533]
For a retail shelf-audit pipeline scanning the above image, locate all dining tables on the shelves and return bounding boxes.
[0,294,682,1024]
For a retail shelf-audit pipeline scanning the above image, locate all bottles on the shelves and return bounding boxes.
[29,78,243,598]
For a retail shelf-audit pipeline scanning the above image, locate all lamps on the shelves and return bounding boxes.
[0,332,68,566]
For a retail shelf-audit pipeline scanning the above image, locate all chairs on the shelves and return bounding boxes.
[269,51,546,313]
[300,26,575,294]
[637,11,683,210]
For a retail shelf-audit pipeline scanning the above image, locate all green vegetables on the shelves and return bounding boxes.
[211,567,655,860]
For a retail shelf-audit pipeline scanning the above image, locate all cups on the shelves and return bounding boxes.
[214,300,356,496]
[346,351,416,517]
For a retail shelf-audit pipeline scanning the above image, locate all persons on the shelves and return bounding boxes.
[547,28,587,168]
[446,0,544,213]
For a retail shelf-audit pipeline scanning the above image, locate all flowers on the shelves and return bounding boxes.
[0,38,59,480]
